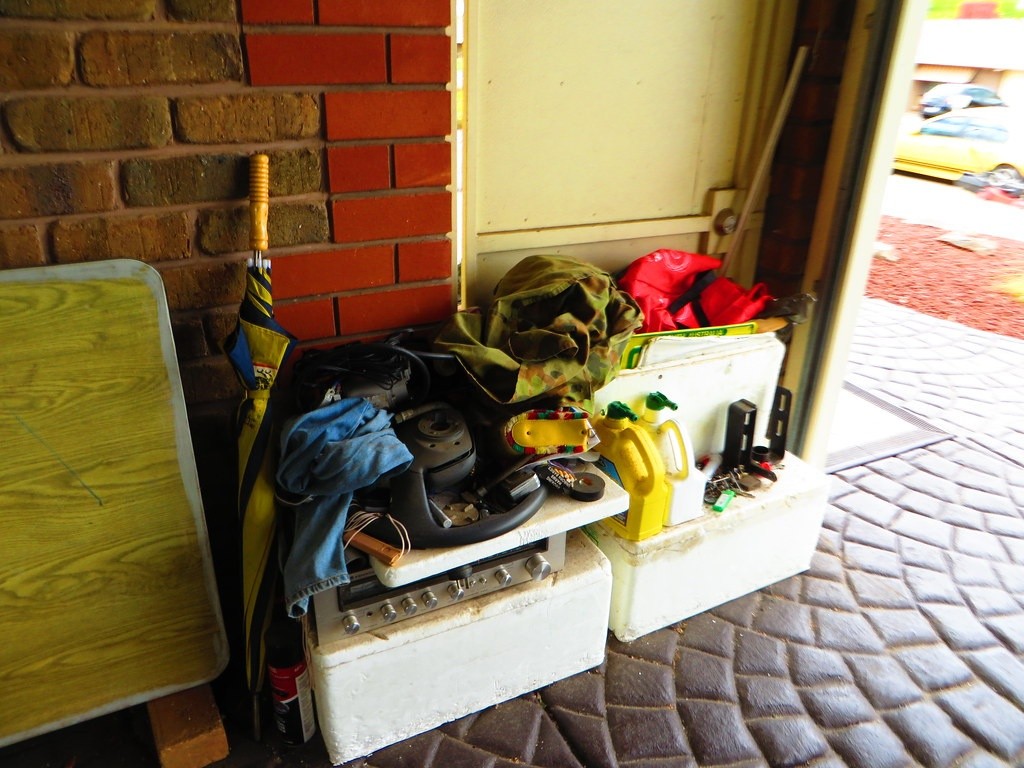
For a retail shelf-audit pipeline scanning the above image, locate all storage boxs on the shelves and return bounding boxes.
[581,448,836,644]
[303,531,614,764]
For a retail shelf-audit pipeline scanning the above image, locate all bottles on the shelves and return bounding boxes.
[589,390,705,539]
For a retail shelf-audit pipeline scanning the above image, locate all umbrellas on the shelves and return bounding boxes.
[223,149,305,759]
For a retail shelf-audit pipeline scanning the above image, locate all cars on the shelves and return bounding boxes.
[892,108,1024,196]
[917,85,1008,120]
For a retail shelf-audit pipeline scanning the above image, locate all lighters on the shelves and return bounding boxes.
[712,487,736,513]
